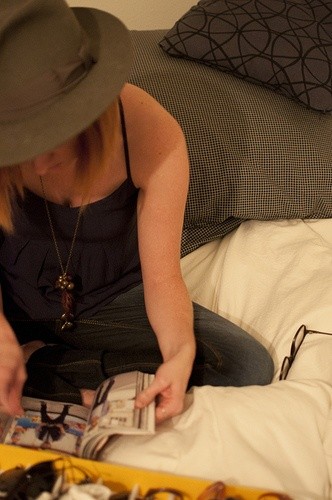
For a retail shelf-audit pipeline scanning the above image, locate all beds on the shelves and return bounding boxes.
[0,32,332,500]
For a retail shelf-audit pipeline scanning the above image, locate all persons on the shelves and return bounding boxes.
[87,376,115,432]
[0,0,277,427]
[35,400,73,449]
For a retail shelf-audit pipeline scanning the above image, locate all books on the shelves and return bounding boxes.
[0,370,157,460]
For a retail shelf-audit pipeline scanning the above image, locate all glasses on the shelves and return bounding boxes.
[280,324,332,381]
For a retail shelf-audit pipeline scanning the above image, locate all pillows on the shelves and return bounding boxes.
[159,0,332,113]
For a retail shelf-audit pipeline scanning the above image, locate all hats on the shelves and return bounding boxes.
[0,0,133,170]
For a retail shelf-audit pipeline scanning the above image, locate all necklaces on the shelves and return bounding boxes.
[40,149,98,329]
